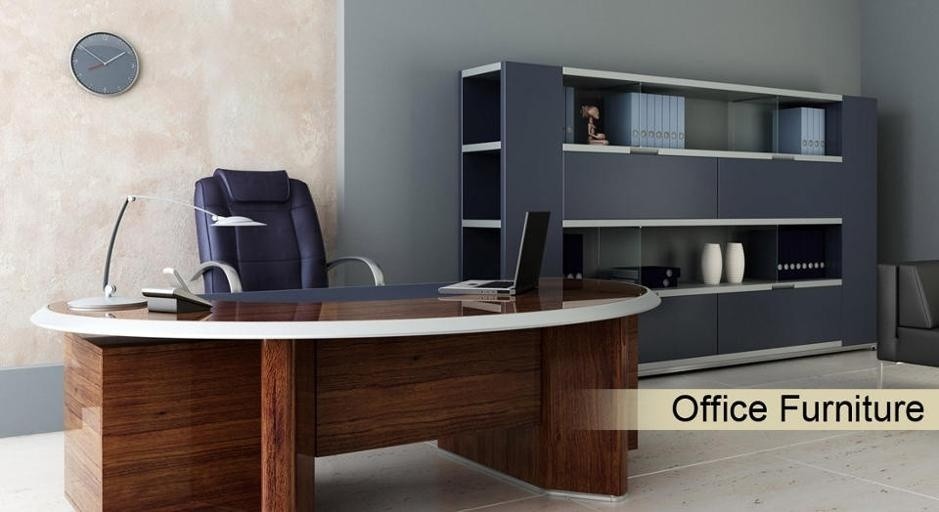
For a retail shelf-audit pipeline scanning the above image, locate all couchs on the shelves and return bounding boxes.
[876,256,938,364]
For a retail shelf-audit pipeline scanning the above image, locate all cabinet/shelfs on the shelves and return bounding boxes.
[456,58,885,383]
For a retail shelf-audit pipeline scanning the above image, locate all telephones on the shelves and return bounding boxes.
[141,268,214,314]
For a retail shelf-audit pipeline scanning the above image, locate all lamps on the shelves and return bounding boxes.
[66,193,268,315]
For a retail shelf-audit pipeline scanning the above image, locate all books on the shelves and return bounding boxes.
[771,107,825,155]
[563,85,576,143]
[609,266,682,288]
[563,232,584,280]
[736,227,829,280]
[603,91,686,149]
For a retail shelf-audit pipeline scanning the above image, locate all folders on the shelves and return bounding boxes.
[563,234,584,280]
[608,266,680,289]
[777,226,825,281]
[603,91,686,148]
[778,107,826,155]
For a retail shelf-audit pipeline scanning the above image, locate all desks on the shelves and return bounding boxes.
[27,277,661,512]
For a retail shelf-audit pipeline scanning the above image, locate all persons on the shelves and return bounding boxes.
[584,106,609,144]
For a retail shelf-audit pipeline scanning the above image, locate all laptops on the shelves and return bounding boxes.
[438,294,541,313]
[437,212,551,294]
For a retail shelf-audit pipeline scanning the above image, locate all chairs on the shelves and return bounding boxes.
[185,166,388,295]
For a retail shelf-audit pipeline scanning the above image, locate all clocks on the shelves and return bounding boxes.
[70,30,141,97]
